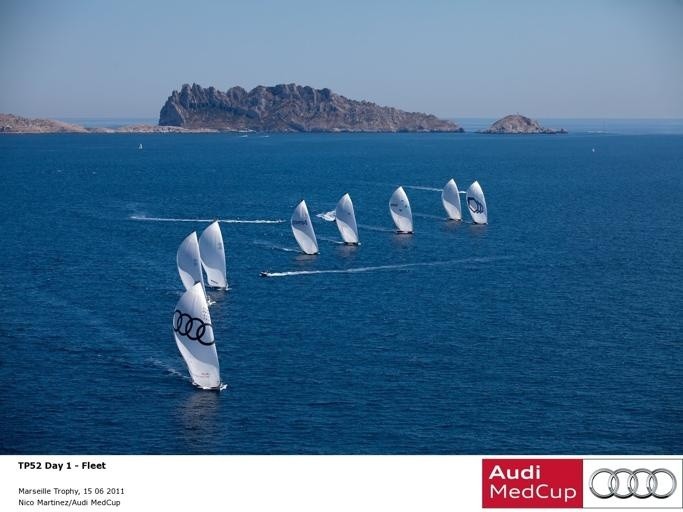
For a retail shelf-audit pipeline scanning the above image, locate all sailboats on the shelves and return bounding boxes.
[389,186,414,233]
[441,179,463,222]
[465,180,488,225]
[176,220,230,306]
[291,200,321,255]
[335,193,361,246]
[171,281,227,391]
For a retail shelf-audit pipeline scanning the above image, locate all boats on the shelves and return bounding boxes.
[260,271,268,277]
